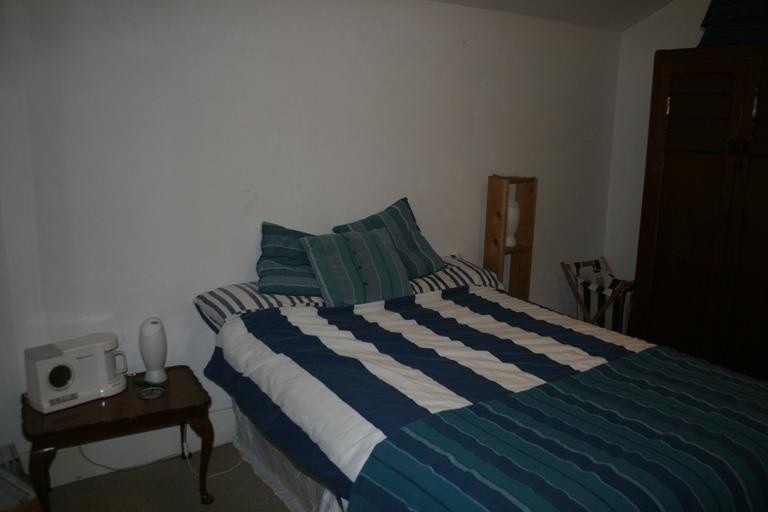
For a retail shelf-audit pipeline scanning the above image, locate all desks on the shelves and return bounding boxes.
[21,366,215,512]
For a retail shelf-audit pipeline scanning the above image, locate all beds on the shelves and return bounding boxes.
[193,253,768,512]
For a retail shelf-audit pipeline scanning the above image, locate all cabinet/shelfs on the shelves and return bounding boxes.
[630,50,768,383]
[487,175,537,304]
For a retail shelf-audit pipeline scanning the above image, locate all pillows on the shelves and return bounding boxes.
[300,229,412,306]
[256,221,322,296]
[332,199,445,279]
